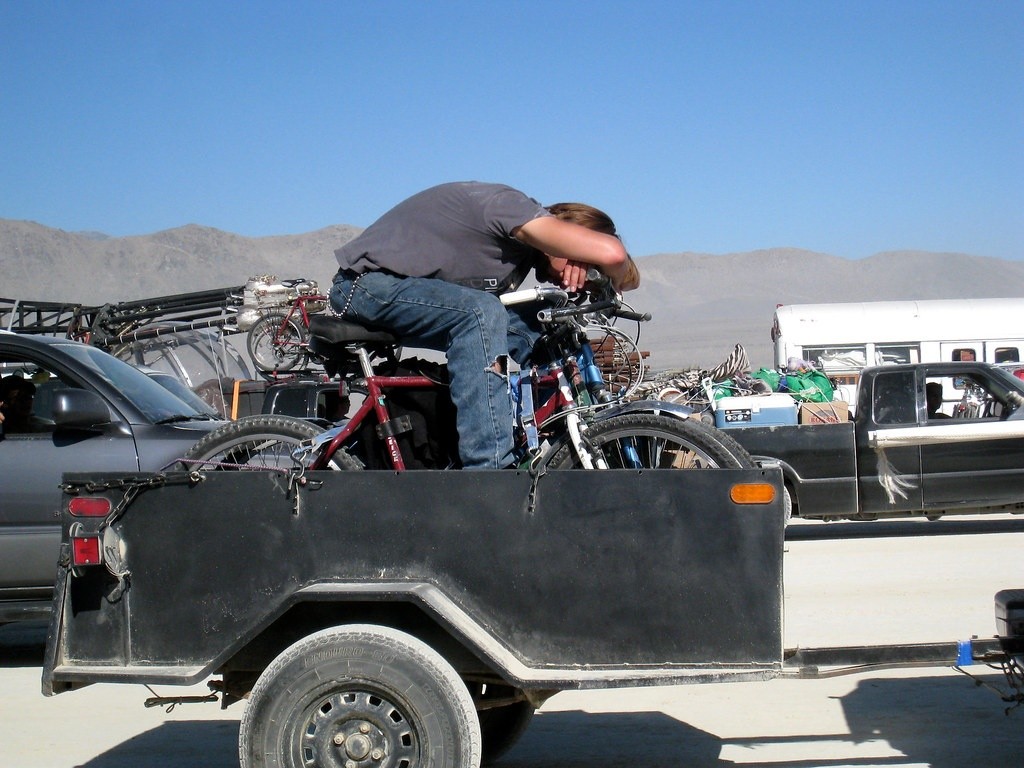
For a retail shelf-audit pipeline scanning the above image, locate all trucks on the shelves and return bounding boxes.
[772,297,1024,416]
[42,468,1023,768]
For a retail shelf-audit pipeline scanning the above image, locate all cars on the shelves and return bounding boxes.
[0,331,327,620]
[0,365,231,425]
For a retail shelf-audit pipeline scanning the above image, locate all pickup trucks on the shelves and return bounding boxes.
[684,362,1024,529]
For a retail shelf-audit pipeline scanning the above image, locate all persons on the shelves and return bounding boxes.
[926,382,952,419]
[330,181,640,470]
[0,375,56,434]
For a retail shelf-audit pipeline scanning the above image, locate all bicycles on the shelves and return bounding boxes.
[179,270,757,469]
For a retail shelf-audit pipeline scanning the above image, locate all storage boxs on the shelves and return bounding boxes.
[712,390,800,427]
[798,402,848,425]
